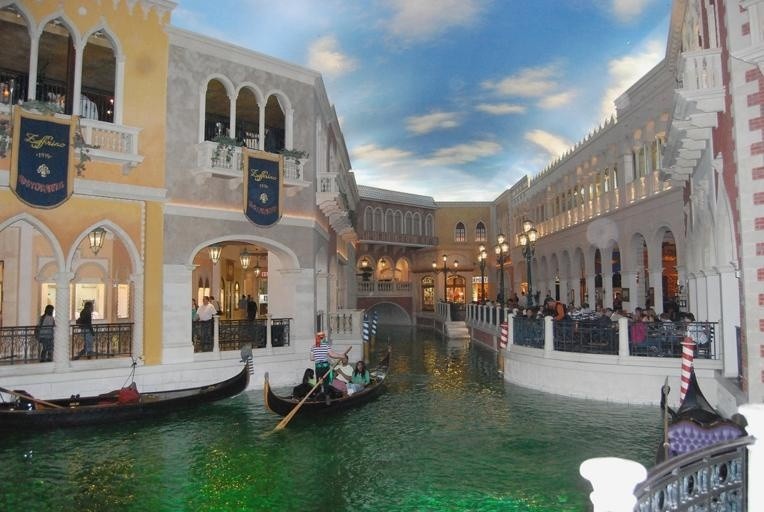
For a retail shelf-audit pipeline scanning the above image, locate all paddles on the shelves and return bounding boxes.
[665,377,668,463]
[273,345,353,430]
[1,386,63,408]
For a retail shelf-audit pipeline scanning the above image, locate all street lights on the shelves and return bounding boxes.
[516,219,538,306]
[494,232,510,303]
[476,243,487,305]
[431,254,459,302]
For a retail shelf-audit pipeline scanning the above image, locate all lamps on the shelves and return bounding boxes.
[361,257,369,268]
[379,257,387,268]
[87,226,108,252]
[239,247,252,270]
[208,243,224,265]
[253,250,263,278]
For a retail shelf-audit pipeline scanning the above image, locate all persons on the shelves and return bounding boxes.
[346,360,370,396]
[331,353,354,395]
[191,293,258,352]
[32,304,55,362]
[471,291,711,357]
[73,300,94,360]
[308,330,348,391]
[302,367,317,387]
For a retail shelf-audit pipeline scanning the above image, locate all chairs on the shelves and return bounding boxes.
[468,292,714,357]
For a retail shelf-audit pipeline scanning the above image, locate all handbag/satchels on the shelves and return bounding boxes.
[119,388,140,403]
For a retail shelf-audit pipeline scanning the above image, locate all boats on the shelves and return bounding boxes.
[651,364,744,498]
[261,336,393,421]
[0,360,255,430]
